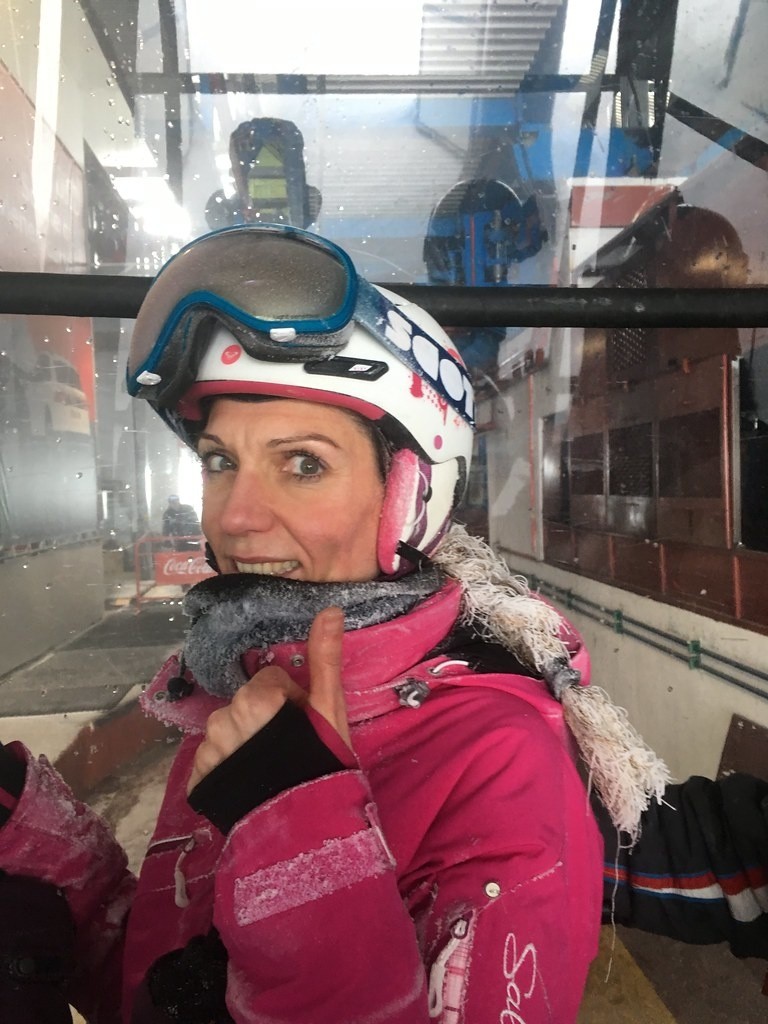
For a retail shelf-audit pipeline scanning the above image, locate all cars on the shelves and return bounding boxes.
[19,350,92,439]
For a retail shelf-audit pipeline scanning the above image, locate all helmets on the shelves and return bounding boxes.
[155,279,475,511]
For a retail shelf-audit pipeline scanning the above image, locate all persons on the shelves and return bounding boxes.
[162,495,205,595]
[0,225,604,1024]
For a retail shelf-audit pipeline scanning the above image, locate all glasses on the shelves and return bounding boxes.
[126,222,360,405]
